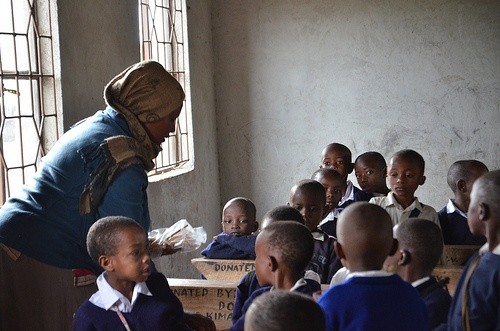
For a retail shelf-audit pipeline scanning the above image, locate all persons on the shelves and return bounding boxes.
[71,216,193,331]
[0,60,184,331]
[201,143,490,286]
[318,201,428,331]
[447,169,500,331]
[383,218,452,331]
[244,289,326,331]
[229,221,314,331]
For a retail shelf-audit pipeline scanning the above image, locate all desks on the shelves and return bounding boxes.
[167,245,480,331]
